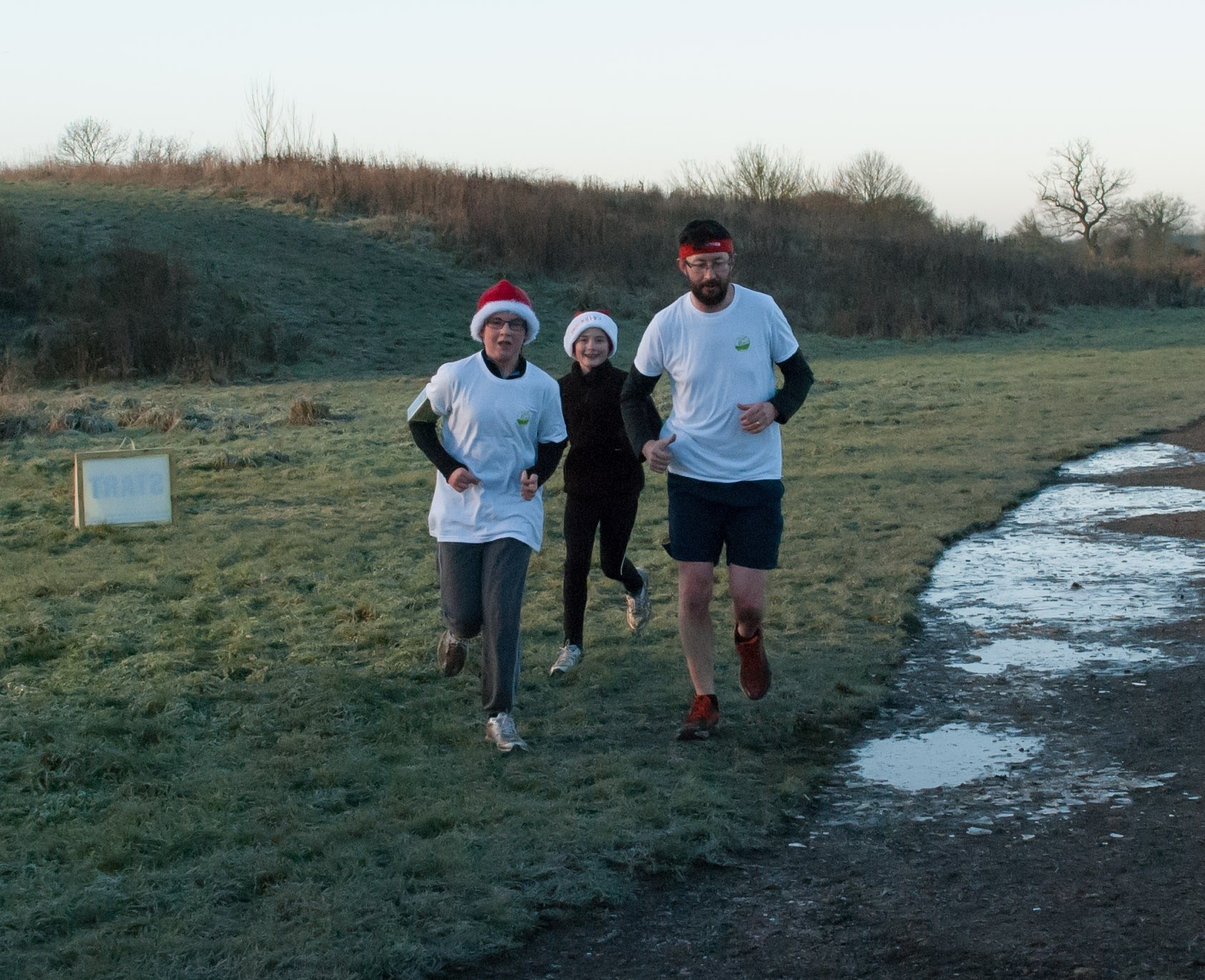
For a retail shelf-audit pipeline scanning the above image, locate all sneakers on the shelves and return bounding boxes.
[733,624,772,700]
[672,692,720,740]
[436,628,468,679]
[484,714,530,757]
[548,640,584,678]
[625,566,653,632]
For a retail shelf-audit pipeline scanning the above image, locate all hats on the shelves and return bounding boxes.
[467,278,541,346]
[561,307,618,361]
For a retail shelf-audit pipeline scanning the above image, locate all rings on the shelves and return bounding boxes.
[755,421,763,426]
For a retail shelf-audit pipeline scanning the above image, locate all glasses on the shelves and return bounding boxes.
[484,317,527,331]
[681,257,734,273]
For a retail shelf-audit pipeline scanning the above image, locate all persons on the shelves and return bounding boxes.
[546,309,664,677]
[406,278,572,755]
[618,216,815,742]
